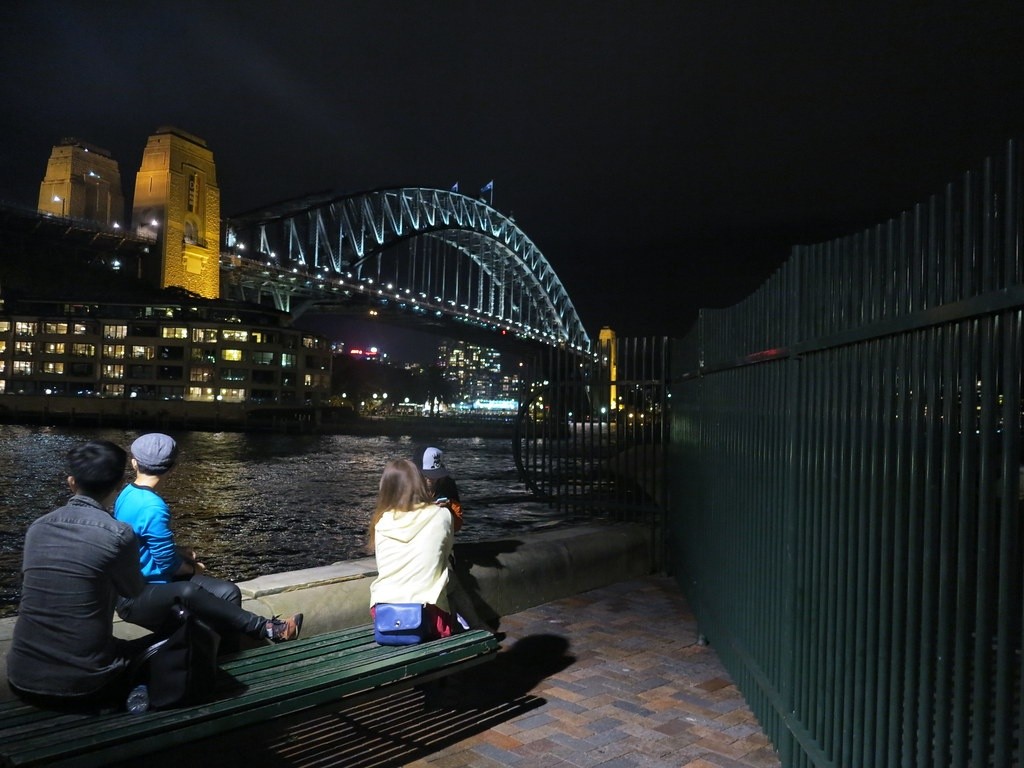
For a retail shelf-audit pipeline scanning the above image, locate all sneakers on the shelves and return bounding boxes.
[264,612,303,643]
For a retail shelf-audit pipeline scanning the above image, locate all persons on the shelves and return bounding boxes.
[112,432,304,655]
[6,440,150,709]
[365,458,506,643]
[421,447,463,533]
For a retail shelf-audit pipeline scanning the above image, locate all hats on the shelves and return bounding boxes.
[131,433,178,471]
[422,447,442,471]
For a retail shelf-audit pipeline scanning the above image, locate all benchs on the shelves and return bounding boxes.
[0,621,500,768]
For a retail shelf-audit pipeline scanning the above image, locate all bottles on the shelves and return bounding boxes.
[126,685,150,713]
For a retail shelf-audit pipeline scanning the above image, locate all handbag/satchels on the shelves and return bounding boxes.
[373,602,426,646]
